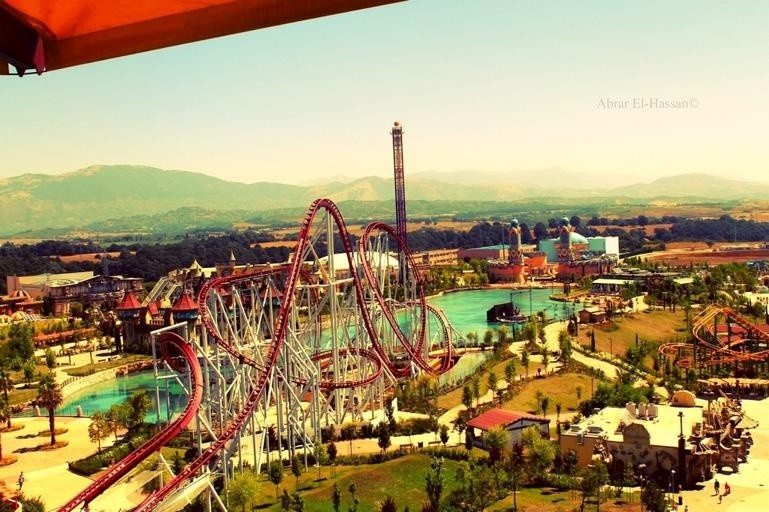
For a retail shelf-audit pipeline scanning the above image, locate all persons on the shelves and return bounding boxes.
[19,471,25,490]
[725,481,730,493]
[714,479,719,494]
[684,505,688,512]
[719,495,722,503]
